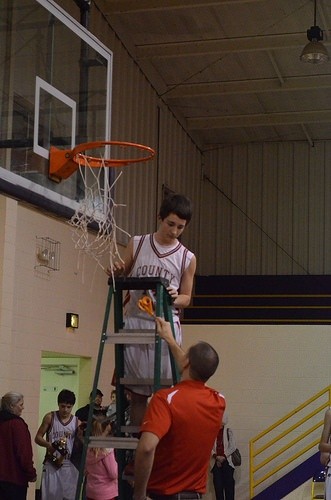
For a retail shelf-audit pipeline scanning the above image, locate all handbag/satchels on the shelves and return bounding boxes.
[227,428,242,466]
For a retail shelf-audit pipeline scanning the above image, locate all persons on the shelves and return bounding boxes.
[106,193,198,429]
[75,389,107,421]
[132,317,226,500]
[35,389,81,500]
[86,420,118,500]
[0,390,38,500]
[317,405,331,500]
[106,390,130,436]
[209,410,241,500]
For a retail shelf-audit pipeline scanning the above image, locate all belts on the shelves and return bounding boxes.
[170,493,198,499]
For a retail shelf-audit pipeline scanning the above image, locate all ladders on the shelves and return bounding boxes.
[73,274,186,500]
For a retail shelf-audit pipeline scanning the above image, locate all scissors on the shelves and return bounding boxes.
[134,295,163,325]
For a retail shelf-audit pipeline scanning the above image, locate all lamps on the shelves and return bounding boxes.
[300,25,331,66]
[66,313,79,328]
